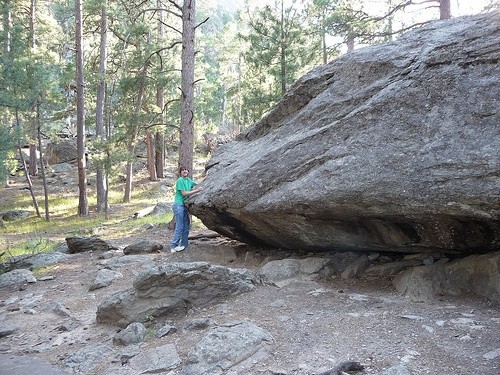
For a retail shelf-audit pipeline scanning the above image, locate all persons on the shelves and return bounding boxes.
[169,167,211,253]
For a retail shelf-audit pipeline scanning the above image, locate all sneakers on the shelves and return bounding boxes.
[171,245,185,254]
